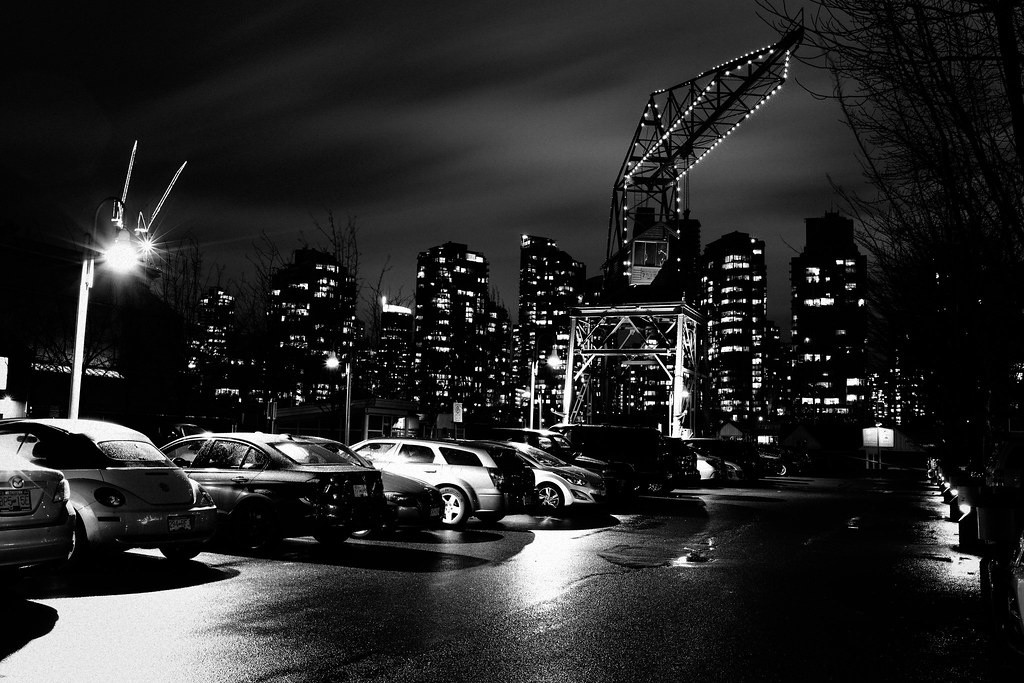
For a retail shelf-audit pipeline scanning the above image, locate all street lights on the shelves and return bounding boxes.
[67,198,154,420]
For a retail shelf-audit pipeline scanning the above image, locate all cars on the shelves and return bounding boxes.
[1,443,81,597]
[1,417,219,562]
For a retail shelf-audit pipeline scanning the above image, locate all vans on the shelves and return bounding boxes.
[155,423,768,553]
